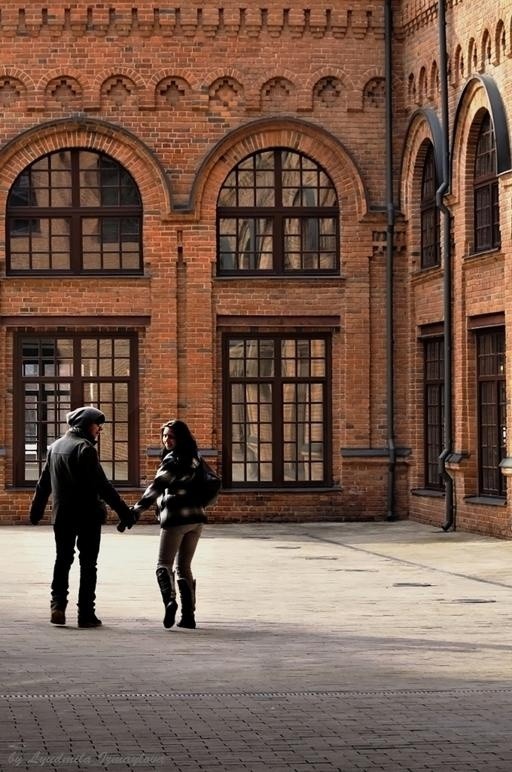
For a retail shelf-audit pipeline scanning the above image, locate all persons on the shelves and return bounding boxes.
[117,420,207,628]
[29,406,133,628]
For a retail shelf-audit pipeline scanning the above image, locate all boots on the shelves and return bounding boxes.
[50,600,68,625]
[77,608,101,628]
[177,578,196,629]
[156,566,178,628]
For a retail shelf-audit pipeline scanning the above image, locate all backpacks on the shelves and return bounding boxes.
[181,454,220,508]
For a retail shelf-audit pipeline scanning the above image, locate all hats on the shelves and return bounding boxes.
[65,407,106,425]
